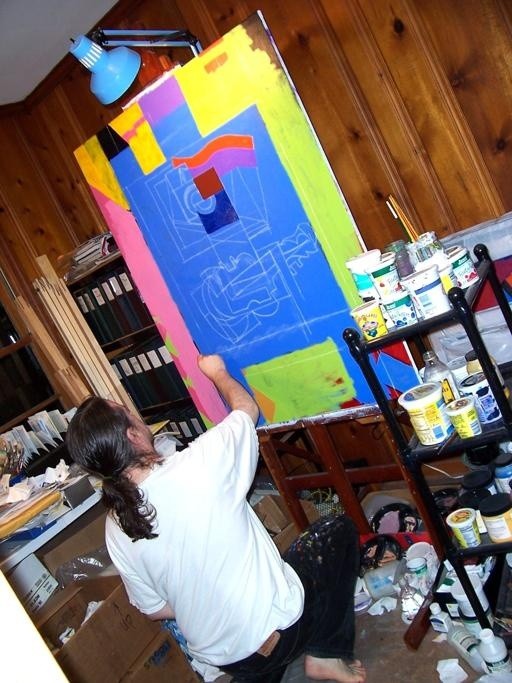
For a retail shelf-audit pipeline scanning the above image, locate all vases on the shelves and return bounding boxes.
[0,472,10,496]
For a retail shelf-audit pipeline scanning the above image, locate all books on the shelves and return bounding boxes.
[1,480,72,534]
[71,230,123,266]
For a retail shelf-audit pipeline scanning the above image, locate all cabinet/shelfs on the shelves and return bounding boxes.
[60,232,206,454]
[339,247,512,633]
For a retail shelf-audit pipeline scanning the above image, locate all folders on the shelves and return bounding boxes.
[110,332,191,408]
[72,266,155,345]
[146,402,206,451]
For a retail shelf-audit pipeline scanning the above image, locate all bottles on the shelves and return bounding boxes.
[421,349,461,409]
[429,601,512,675]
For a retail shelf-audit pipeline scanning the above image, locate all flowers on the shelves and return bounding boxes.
[0,436,25,476]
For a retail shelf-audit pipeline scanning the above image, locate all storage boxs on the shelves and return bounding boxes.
[415,209,512,315]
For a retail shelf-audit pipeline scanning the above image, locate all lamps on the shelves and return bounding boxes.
[67,25,202,107]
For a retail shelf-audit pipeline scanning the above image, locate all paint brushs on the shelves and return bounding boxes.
[386,194,430,262]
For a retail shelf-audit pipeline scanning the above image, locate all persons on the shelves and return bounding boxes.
[64,349,369,682]
